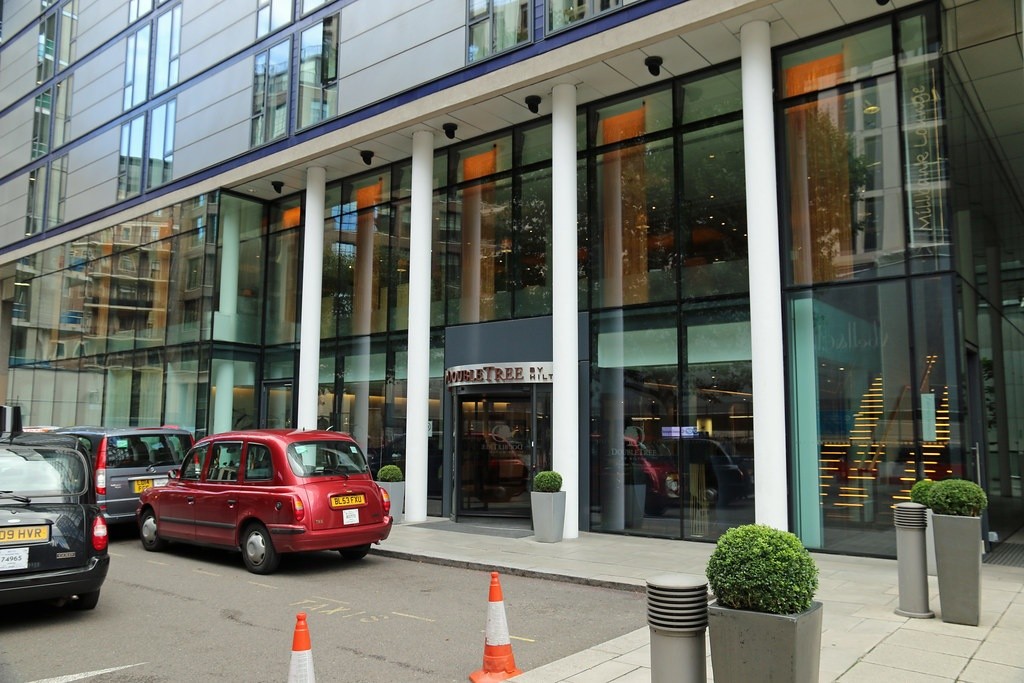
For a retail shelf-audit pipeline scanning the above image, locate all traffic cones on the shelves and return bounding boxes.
[467,570,524,683]
[286,611,317,683]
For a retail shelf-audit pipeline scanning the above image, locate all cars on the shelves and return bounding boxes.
[661,436,751,509]
[136,427,394,575]
[589,432,682,515]
[378,428,528,502]
[49,424,200,526]
[0,430,111,611]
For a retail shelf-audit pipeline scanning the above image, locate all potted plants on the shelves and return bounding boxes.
[530,472,567,543]
[928,479,989,626]
[707,523,824,683]
[375,465,405,525]
[910,480,938,576]
